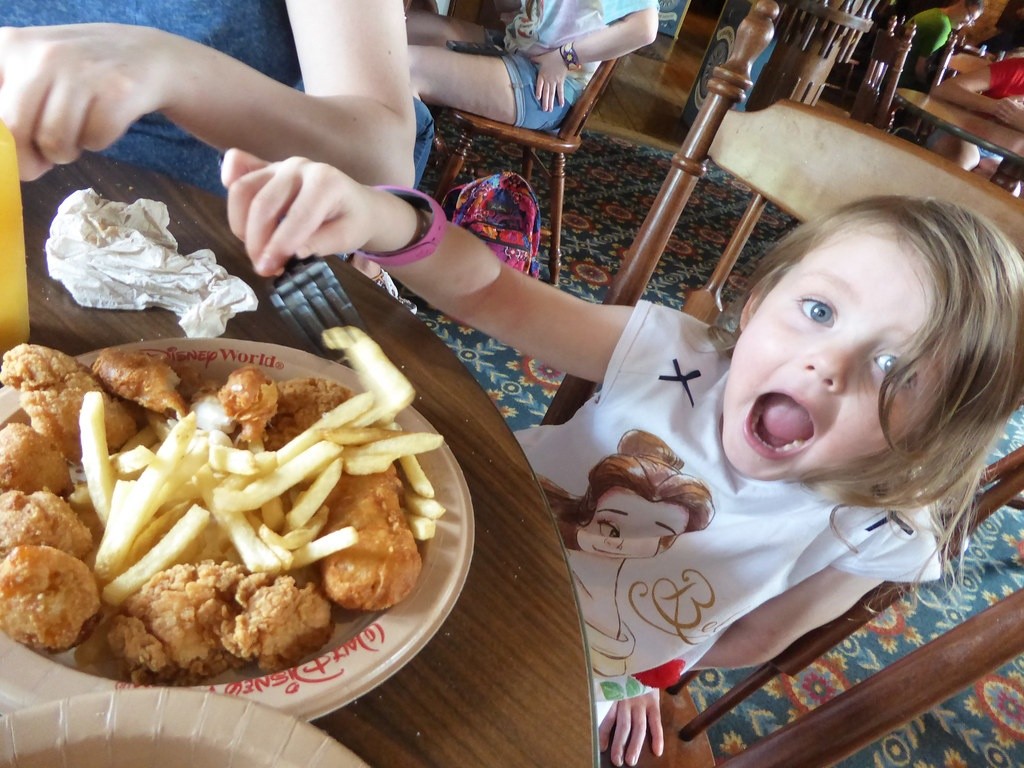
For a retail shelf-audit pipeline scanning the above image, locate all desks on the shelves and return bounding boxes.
[895,88,1024,194]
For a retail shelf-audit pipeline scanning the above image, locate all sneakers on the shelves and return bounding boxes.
[369,268,417,315]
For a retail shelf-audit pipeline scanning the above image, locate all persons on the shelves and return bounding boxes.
[0,0,418,202]
[221,148,1024,768]
[405,0,662,134]
[896,0,1024,199]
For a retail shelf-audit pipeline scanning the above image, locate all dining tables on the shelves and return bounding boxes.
[0,150,597,767]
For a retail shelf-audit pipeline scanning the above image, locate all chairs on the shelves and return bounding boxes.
[424,0,1024,768]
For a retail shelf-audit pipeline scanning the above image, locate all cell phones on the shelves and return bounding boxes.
[446,40,508,56]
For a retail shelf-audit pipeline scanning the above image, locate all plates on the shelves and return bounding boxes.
[0,338,476,719]
[1,686,370,768]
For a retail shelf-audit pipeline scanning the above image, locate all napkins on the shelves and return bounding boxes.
[45,188,259,340]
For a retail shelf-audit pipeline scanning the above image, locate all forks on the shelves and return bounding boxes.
[264,254,366,357]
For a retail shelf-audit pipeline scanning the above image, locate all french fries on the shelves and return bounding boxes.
[73,326,445,664]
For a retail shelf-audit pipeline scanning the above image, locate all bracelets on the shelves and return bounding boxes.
[565,42,580,71]
[356,185,448,267]
[559,46,565,61]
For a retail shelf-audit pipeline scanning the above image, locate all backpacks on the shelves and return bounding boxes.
[440,171,541,280]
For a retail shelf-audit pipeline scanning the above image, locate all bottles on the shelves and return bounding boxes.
[1,118,29,352]
